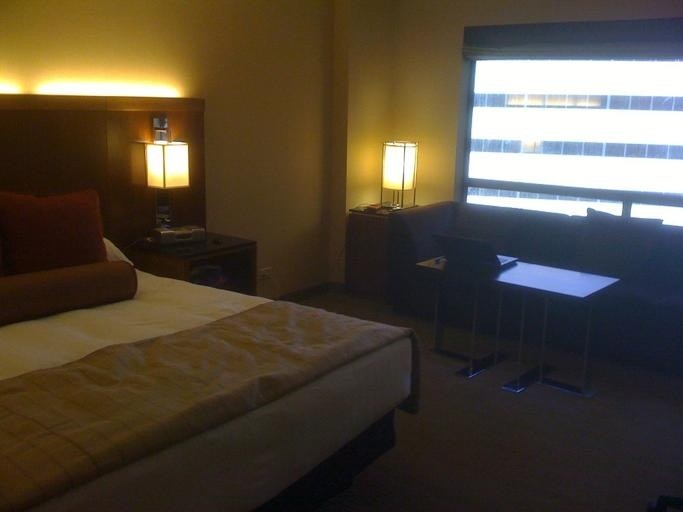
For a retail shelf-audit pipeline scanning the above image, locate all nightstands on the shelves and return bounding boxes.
[126,233,257,300]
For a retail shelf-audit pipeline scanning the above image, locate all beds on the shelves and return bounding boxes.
[0,268,412,512]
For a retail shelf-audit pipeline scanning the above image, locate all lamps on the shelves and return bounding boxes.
[144,140,191,228]
[381,139,419,211]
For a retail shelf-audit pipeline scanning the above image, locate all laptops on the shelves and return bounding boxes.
[432,234,519,270]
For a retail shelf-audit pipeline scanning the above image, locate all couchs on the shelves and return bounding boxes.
[388,200,682,378]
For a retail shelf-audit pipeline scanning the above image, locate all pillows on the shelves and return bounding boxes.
[0,189,107,277]
[1,262,138,328]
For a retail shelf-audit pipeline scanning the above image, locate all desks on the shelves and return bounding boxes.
[415,254,621,399]
[344,201,418,298]
